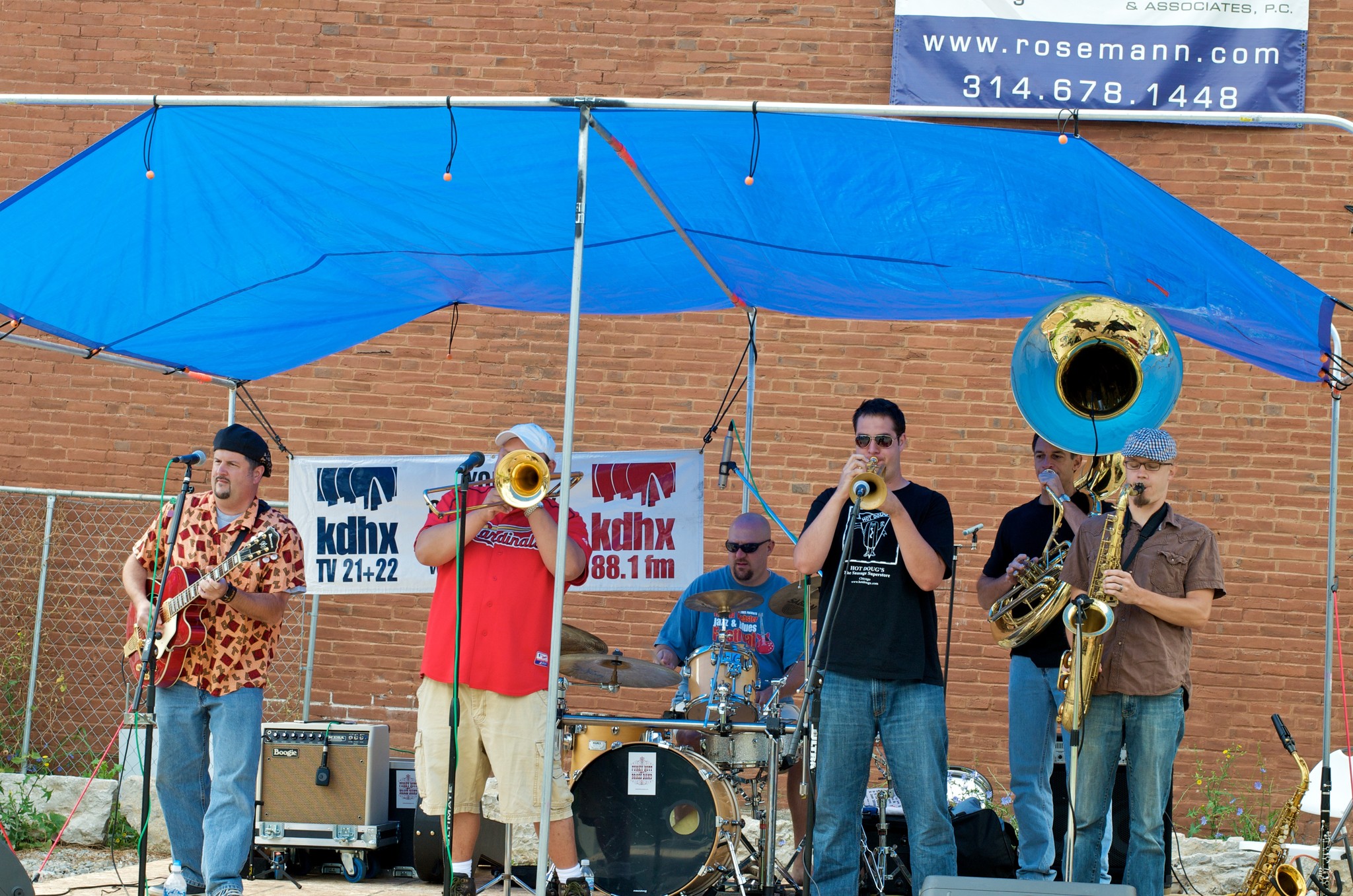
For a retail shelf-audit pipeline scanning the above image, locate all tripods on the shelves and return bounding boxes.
[476,820,536,896]
[702,770,803,896]
[858,746,913,891]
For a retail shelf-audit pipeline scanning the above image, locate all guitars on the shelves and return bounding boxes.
[123,526,283,689]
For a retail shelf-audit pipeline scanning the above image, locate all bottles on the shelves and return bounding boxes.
[580,860,594,896]
[163,860,187,896]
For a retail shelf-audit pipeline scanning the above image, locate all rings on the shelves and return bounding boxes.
[1118,584,1122,592]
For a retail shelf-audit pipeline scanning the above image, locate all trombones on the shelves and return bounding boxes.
[423,449,584,519]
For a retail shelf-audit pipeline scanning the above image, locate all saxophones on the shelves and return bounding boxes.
[1056,481,1146,733]
[1234,713,1306,896]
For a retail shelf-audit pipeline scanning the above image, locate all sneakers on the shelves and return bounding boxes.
[449,871,477,896]
[216,888,244,896]
[148,877,207,896]
[558,876,591,896]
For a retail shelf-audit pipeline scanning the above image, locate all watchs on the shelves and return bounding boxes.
[1055,493,1071,508]
[523,501,544,518]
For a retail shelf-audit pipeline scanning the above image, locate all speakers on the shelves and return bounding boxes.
[920,876,1137,896]
[254,722,391,825]
[0,839,36,896]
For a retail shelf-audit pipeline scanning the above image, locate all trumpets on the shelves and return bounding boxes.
[849,456,887,510]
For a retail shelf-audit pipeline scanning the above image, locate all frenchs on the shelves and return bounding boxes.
[987,292,1183,649]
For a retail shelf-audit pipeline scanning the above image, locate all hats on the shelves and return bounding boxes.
[1121,427,1177,462]
[495,423,556,464]
[213,423,272,478]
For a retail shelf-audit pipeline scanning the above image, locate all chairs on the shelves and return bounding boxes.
[1239,747,1353,896]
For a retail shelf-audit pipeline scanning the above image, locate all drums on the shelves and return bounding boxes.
[683,643,759,737]
[570,712,670,792]
[569,742,745,896]
[699,733,803,769]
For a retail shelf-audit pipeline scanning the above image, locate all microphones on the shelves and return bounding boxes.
[316,737,330,786]
[171,450,206,466]
[963,523,984,535]
[455,451,485,474]
[853,480,870,498]
[718,421,734,490]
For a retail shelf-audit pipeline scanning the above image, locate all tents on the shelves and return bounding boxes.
[0,97,1353,896]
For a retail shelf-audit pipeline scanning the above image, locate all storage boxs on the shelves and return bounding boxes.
[294,761,419,879]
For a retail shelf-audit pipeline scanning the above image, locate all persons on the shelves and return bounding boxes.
[1038,428,1230,896]
[414,424,595,896]
[977,434,1112,884]
[122,425,307,894]
[794,398,956,896]
[654,512,812,896]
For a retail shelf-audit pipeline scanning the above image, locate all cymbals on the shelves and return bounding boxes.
[559,649,683,688]
[559,622,608,654]
[768,576,823,620]
[684,589,764,614]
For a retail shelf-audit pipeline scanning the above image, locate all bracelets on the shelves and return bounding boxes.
[220,582,237,603]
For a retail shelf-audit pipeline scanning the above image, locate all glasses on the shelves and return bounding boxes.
[1123,461,1173,472]
[854,434,900,449]
[726,538,772,553]
